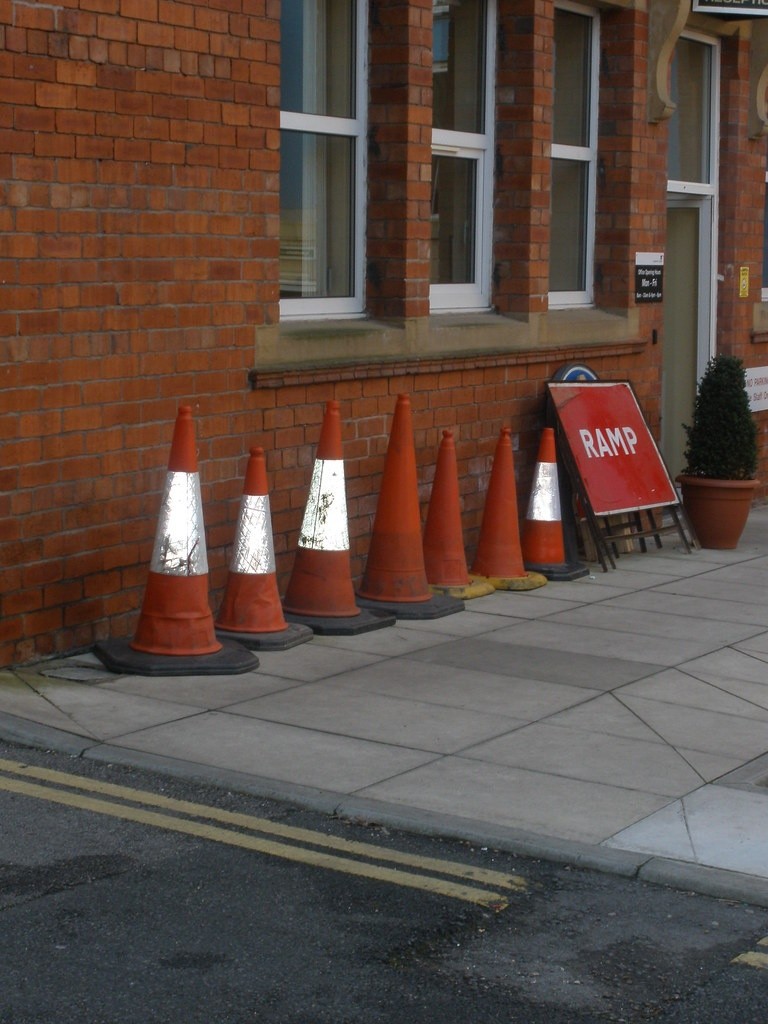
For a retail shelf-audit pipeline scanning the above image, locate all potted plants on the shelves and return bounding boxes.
[675,353,760,550]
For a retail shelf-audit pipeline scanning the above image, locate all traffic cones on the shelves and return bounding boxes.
[421,430,495,601]
[90,405,261,677]
[353,391,466,621]
[519,427,590,581]
[211,444,314,652]
[467,425,549,590]
[280,397,397,636]
